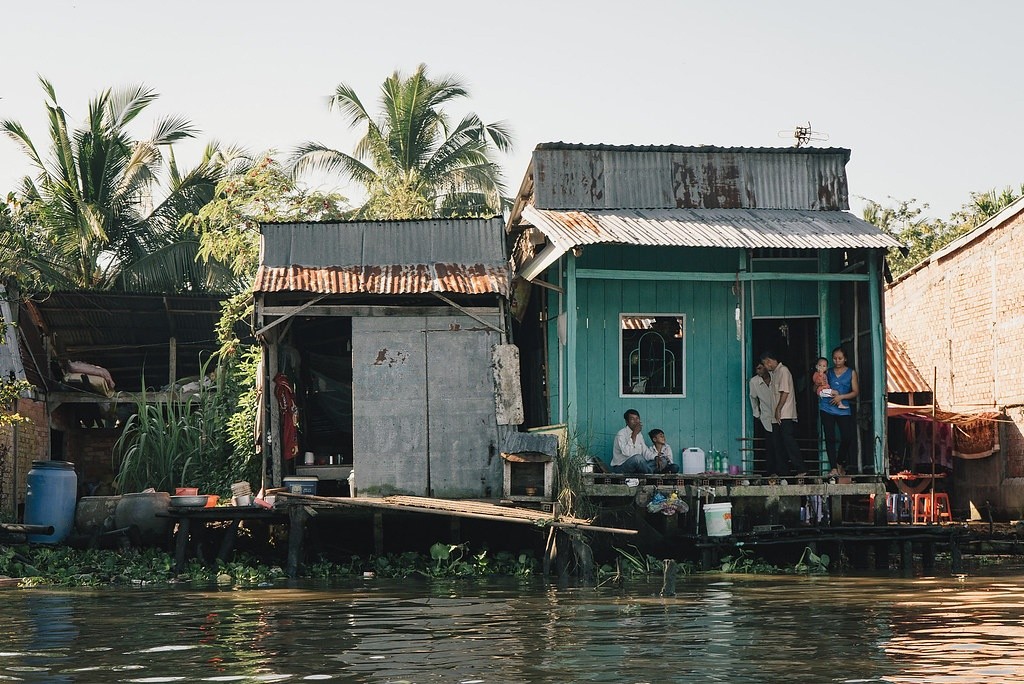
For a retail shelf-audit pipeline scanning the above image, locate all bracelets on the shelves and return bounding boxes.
[655,456,659,461]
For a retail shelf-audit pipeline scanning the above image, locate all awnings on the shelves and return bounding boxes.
[521,204,910,258]
[253,263,509,299]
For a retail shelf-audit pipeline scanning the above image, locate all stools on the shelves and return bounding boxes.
[869,492,952,523]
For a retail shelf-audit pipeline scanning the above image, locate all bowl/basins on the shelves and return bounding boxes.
[169,495,220,506]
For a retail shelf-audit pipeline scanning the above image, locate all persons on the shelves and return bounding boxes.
[813,346,860,475]
[609,409,667,474]
[749,351,810,475]
[813,357,849,410]
[648,429,679,474]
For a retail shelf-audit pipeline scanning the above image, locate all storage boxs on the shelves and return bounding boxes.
[283,476,319,495]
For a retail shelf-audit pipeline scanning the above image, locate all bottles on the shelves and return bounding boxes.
[722,451,729,473]
[706,450,714,472]
[715,451,721,474]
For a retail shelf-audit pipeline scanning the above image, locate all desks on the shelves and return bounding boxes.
[889,473,947,522]
[296,464,353,496]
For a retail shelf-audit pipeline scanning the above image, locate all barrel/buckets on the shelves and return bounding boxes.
[682,447,705,474]
[23,460,78,544]
[703,503,732,537]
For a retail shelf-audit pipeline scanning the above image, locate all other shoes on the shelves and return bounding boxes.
[826,469,838,476]
[836,463,842,476]
